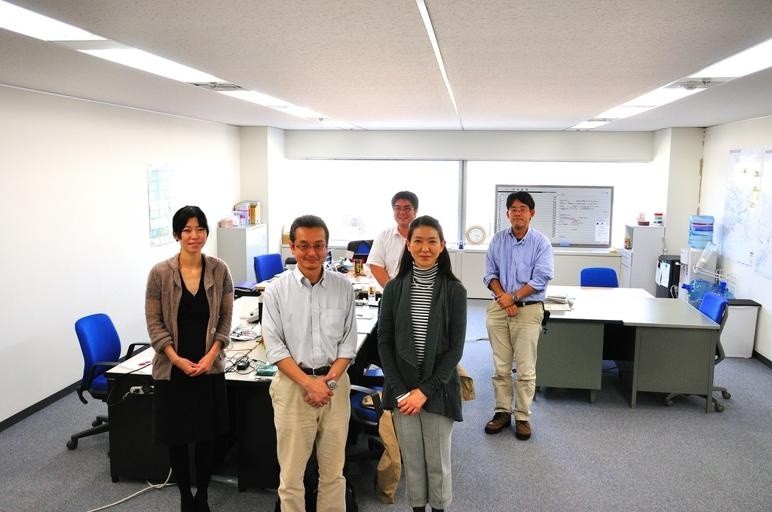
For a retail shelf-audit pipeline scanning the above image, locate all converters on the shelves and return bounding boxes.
[237,360,250,370]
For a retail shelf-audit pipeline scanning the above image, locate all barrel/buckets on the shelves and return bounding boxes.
[688,215,715,249]
[699,282,735,300]
[682,278,719,303]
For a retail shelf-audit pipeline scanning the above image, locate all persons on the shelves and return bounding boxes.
[375,211,468,510]
[480,189,555,439]
[361,187,427,291]
[145,204,235,511]
[259,214,361,512]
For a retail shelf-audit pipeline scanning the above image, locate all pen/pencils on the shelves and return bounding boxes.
[356,314,363,317]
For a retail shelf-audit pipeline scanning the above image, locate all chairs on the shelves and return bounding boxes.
[350,364,386,455]
[68,314,157,455]
[579,266,621,289]
[253,252,284,281]
[659,292,736,413]
[347,238,374,262]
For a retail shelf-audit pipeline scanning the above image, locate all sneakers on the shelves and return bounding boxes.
[485,409,512,435]
[515,417,532,441]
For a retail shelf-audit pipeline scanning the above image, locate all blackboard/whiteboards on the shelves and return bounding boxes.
[494,184,614,248]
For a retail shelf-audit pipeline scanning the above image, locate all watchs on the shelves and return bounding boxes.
[509,292,520,303]
[325,378,338,392]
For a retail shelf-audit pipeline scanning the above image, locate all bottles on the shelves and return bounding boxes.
[355,257,364,276]
[239,215,246,224]
[654,211,663,226]
[366,286,378,304]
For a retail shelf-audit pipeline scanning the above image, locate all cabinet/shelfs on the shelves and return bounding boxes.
[618,221,665,297]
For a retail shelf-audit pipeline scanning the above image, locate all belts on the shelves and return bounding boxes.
[299,365,331,376]
[514,298,540,308]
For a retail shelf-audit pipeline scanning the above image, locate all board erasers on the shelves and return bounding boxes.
[559,240,571,246]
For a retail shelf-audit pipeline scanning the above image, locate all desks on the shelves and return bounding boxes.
[524,285,721,417]
[107,295,381,481]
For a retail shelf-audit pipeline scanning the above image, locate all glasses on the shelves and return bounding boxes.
[394,205,415,212]
[510,207,531,214]
[293,243,327,249]
